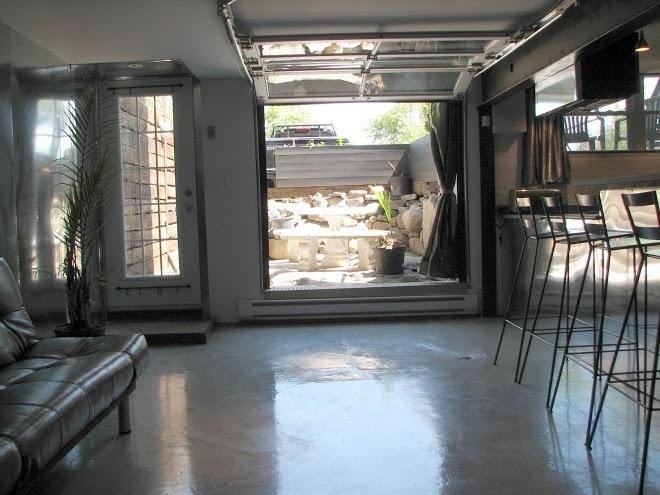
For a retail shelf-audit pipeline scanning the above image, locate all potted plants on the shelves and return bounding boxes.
[367,181,407,274]
[49,72,122,339]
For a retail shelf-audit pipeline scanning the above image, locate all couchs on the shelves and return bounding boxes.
[0,254,149,495]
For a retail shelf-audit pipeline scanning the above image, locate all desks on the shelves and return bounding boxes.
[273,205,397,272]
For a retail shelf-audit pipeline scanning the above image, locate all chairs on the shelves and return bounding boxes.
[562,95,660,153]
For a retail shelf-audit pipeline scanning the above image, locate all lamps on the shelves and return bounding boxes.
[633,22,651,54]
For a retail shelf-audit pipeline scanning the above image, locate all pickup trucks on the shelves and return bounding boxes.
[265,121,350,189]
